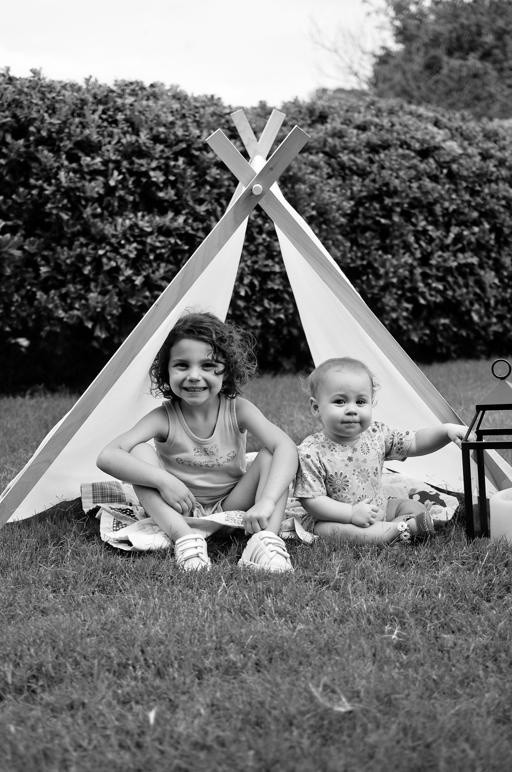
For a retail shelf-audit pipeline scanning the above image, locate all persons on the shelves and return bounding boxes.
[96,312,299,573]
[298,358,479,547]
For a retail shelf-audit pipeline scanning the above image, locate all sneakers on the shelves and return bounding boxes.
[173,534,211,573]
[237,530,295,573]
[397,510,437,543]
